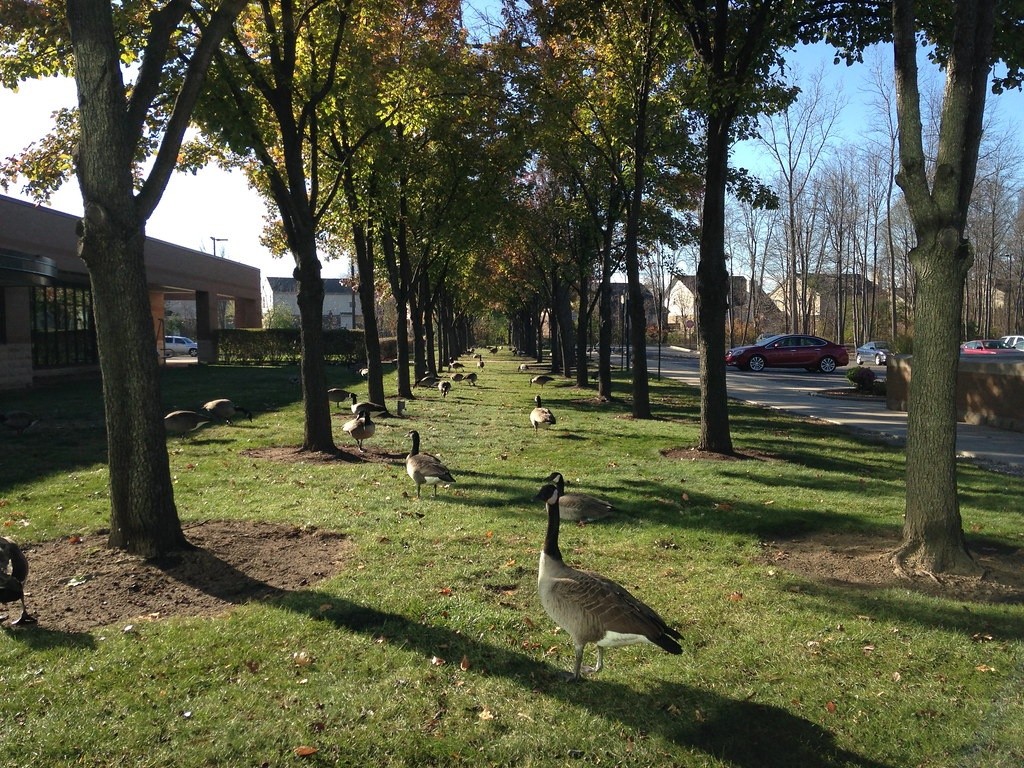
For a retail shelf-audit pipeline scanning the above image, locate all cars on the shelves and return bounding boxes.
[998,335,1024,348]
[754,333,775,344]
[726,335,849,375]
[164,336,198,358]
[856,341,898,366]
[1013,341,1024,353]
[960,340,1021,354]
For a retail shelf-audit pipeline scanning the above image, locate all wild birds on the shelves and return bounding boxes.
[0,536,38,627]
[162,344,684,682]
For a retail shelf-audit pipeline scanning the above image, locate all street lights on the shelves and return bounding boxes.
[210,237,228,256]
